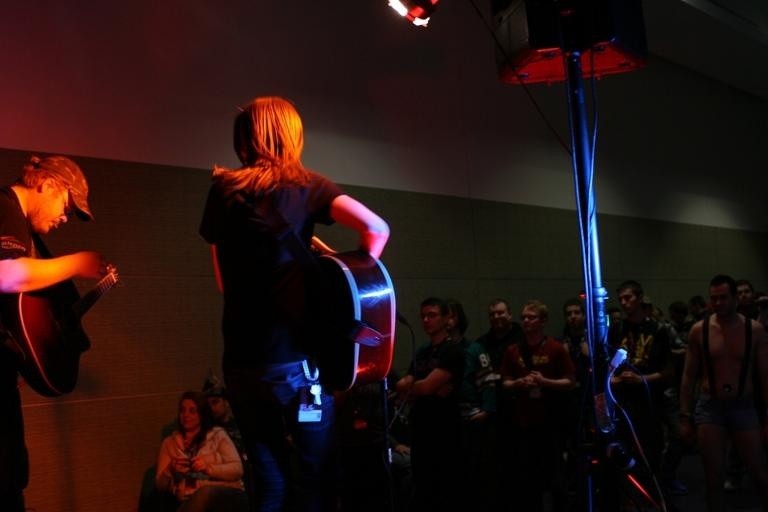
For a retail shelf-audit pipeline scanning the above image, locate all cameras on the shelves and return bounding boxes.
[183,459,195,467]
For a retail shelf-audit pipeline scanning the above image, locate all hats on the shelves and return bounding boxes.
[31,156,95,223]
[203,376,227,396]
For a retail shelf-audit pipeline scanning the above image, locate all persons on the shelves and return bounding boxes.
[197,95,390,512]
[0,155,109,511]
[155,274,767,512]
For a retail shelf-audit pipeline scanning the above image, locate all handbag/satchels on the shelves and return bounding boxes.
[172,472,245,501]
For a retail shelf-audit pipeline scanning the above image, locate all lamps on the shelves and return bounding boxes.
[386,0,441,30]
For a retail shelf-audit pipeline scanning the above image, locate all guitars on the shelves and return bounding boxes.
[13,265,124,397]
[312,236,396,398]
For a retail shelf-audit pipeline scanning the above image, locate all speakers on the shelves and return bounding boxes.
[491,0,647,87]
